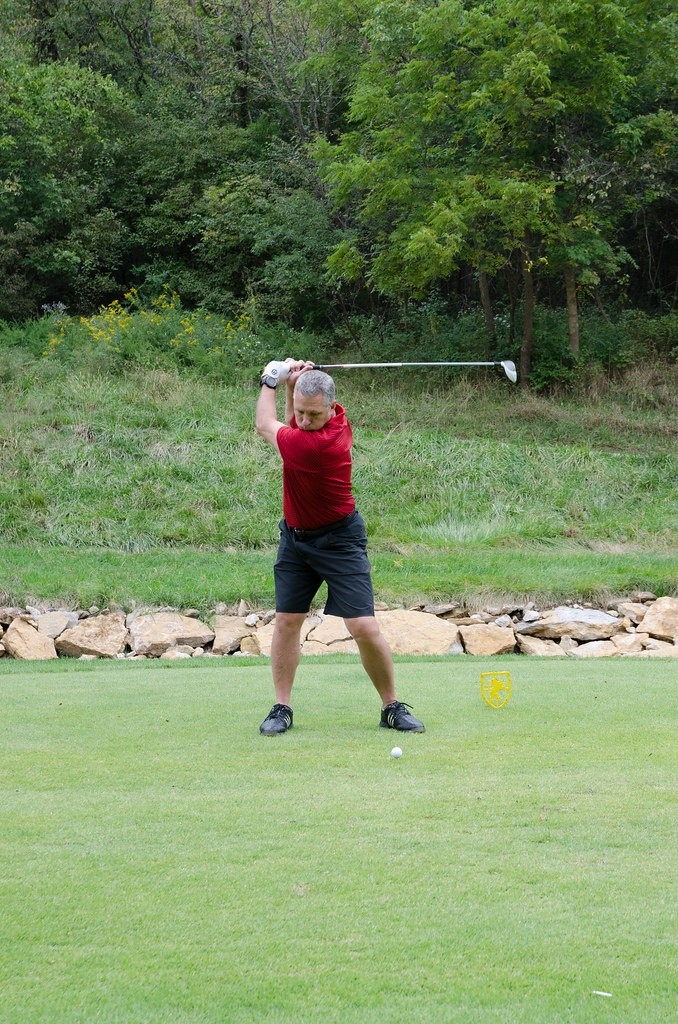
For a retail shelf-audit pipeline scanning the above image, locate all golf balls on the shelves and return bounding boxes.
[391,747,402,758]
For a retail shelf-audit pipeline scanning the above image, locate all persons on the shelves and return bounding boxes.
[255,357,426,735]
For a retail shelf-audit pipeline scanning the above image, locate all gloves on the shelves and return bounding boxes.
[260,360,292,389]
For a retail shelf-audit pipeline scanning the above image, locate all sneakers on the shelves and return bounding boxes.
[379,701,426,734]
[259,703,294,737]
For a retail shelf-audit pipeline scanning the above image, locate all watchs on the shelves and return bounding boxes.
[260,376,277,389]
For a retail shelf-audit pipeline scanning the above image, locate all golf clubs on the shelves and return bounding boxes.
[300,360,517,383]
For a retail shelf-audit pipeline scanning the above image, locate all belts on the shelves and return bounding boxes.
[292,511,355,543]
